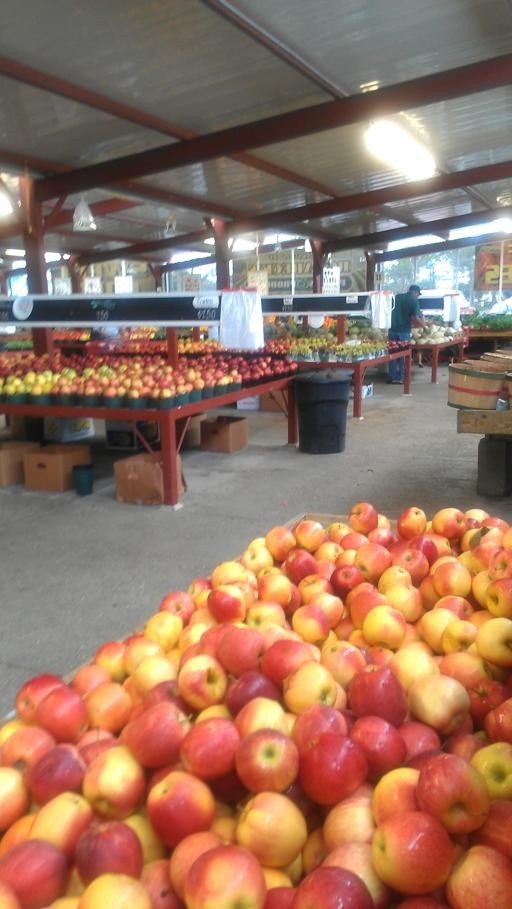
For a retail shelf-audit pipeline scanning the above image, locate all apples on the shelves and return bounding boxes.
[0,350,298,399]
[0,501,512,909]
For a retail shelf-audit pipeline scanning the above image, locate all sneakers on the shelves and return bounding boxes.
[391,380,404,384]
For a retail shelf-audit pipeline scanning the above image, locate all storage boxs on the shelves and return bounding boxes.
[0,390,288,505]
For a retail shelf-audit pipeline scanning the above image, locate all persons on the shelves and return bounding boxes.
[385,284,432,384]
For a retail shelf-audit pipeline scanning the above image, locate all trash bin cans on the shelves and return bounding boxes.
[295,372,351,454]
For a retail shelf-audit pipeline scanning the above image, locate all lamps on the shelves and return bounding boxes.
[72,190,97,232]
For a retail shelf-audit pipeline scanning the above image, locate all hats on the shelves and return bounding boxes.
[409,285,422,295]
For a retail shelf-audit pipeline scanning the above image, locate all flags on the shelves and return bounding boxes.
[473,242,500,291]
[501,239,512,289]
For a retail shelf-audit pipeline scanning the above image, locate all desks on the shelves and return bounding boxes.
[408,339,463,383]
[0,375,298,506]
[297,350,412,417]
